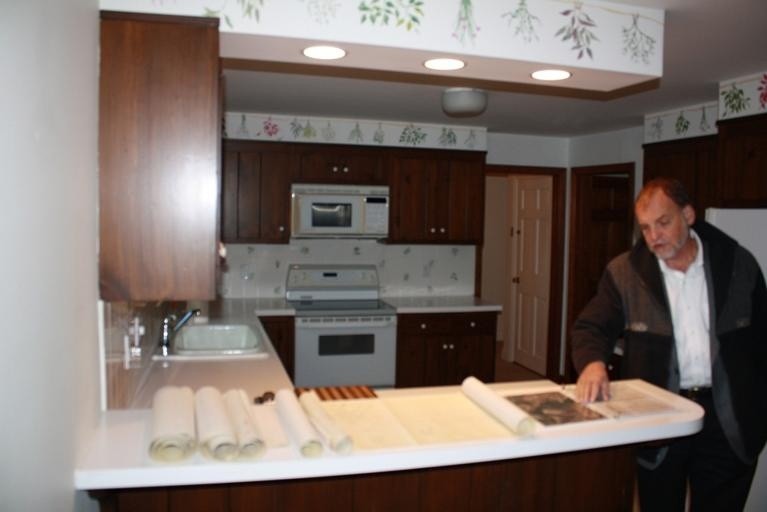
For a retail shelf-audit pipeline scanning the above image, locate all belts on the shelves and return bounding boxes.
[679,388,713,404]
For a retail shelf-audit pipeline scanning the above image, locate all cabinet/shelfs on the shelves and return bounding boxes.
[396,312,498,388]
[377,145,488,246]
[219,139,302,245]
[102,10,224,303]
[260,315,295,387]
[301,143,379,185]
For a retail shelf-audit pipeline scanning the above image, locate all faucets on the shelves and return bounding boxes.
[172,309,201,334]
[110,311,141,349]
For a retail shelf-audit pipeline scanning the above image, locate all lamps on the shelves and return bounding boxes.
[441,86,487,117]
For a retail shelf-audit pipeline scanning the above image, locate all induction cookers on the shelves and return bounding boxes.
[284,300,396,310]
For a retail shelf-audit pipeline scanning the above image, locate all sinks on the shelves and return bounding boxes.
[151,323,269,360]
[102,316,161,356]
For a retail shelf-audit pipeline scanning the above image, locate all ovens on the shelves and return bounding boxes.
[295,315,397,388]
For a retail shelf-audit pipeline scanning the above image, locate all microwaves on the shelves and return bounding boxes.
[291,184,389,239]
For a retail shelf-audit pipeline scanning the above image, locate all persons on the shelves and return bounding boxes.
[569,176,767,512]
